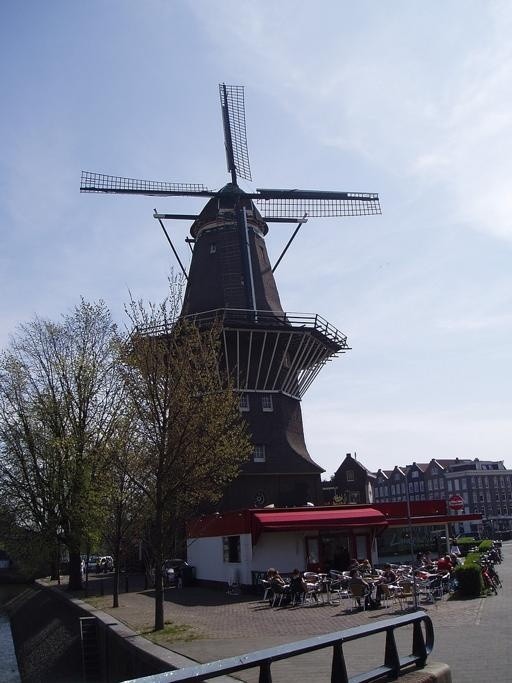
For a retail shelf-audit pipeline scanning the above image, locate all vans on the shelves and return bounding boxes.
[87,555,113,572]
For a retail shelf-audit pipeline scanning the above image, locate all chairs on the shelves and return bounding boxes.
[261,557,463,612]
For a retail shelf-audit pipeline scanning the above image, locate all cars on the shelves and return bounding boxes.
[144,557,192,590]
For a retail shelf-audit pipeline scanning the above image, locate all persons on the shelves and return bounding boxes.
[266,552,461,606]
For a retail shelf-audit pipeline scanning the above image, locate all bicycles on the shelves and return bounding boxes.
[471,537,507,596]
